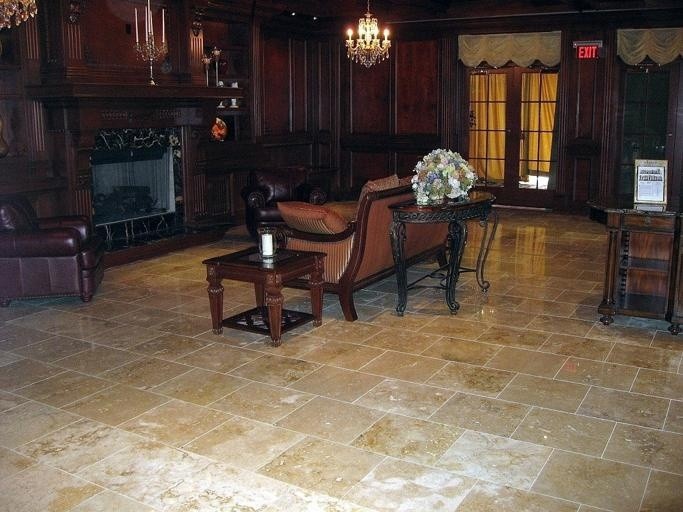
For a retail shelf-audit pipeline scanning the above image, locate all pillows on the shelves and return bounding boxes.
[400,176,414,186]
[323,200,358,222]
[356,174,399,218]
[277,202,348,235]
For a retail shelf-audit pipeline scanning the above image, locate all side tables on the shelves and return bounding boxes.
[202,247,328,347]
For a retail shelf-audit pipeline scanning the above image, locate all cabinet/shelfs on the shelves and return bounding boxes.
[666,211,683,335]
[597,209,681,326]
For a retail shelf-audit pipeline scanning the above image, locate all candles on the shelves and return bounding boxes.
[162,9,165,43]
[262,234,275,257]
[148,0,150,32]
[145,6,148,42]
[150,11,153,35]
[135,7,139,43]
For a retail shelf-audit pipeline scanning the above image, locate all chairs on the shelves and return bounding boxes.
[241,169,328,239]
[0,191,105,306]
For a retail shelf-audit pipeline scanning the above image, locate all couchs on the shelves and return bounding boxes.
[279,186,451,321]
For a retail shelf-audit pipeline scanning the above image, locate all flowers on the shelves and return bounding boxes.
[412,148,478,206]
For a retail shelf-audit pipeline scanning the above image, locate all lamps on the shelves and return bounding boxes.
[344,0,393,68]
[0,0,38,31]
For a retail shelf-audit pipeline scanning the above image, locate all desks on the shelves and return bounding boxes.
[388,192,498,316]
